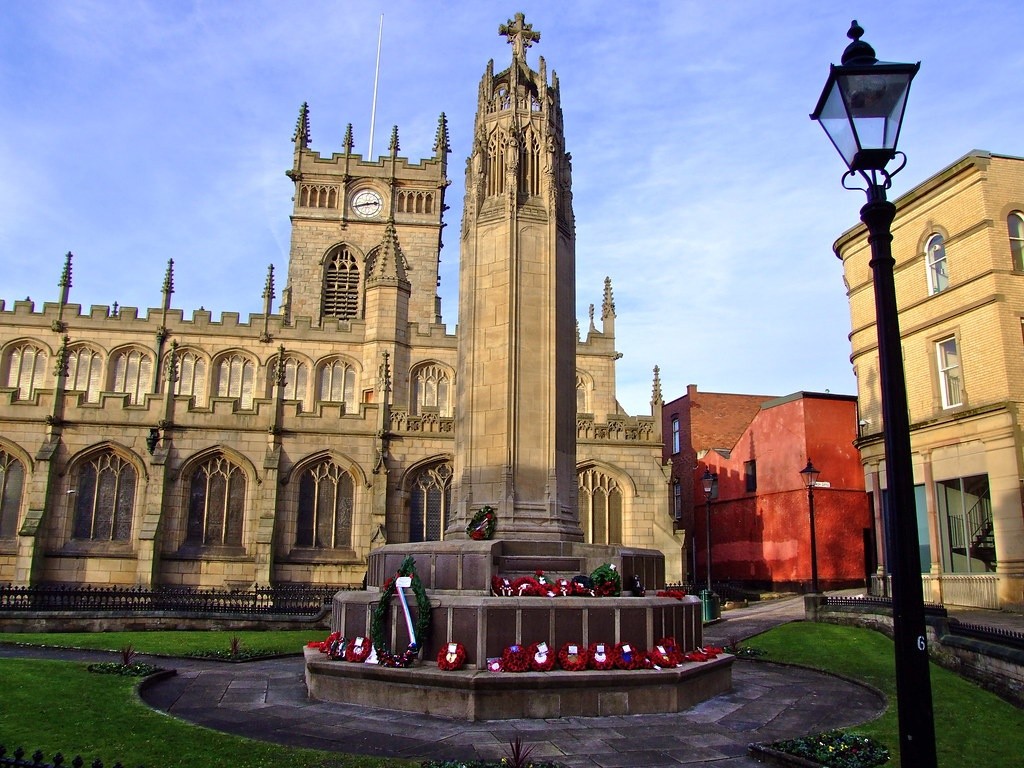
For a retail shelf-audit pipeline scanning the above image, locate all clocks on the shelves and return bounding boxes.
[350,188,385,220]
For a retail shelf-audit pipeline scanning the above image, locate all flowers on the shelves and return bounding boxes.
[503,644,530,672]
[319,632,341,653]
[488,659,504,673]
[639,651,655,669]
[703,645,723,654]
[660,638,682,662]
[693,650,717,659]
[371,554,433,668]
[438,642,465,671]
[493,564,623,597]
[653,646,677,667]
[327,637,349,660]
[527,641,556,672]
[466,506,497,540]
[345,637,371,663]
[587,642,614,671]
[685,654,708,662]
[656,590,685,599]
[613,642,639,670]
[558,642,587,671]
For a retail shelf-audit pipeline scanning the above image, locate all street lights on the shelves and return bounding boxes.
[798,458,822,595]
[700,468,722,619]
[813,19,942,768]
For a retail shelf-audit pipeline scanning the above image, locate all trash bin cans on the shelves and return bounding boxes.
[699,589,714,622]
